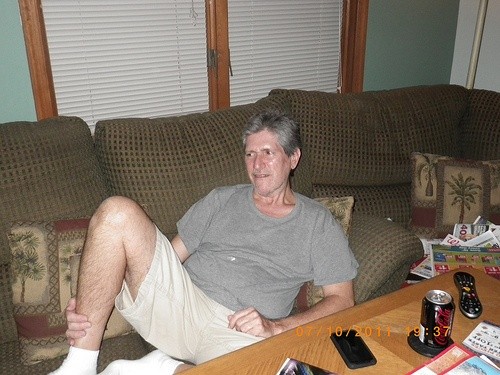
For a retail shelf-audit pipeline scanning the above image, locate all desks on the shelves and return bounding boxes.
[173,267,499,375]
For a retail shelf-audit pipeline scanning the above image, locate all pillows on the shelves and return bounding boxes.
[466,160,500,229]
[5,219,145,366]
[293,196,354,317]
[409,153,490,233]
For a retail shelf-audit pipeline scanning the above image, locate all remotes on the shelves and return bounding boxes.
[453,272,482,319]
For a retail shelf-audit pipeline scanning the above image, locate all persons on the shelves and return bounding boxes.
[45,109,359,375]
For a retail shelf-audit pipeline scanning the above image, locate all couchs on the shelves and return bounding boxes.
[269,85,500,237]
[1,103,423,374]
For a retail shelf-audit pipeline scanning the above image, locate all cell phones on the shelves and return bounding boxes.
[330,328,377,369]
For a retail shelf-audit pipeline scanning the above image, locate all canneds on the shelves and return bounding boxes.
[418,289,455,348]
